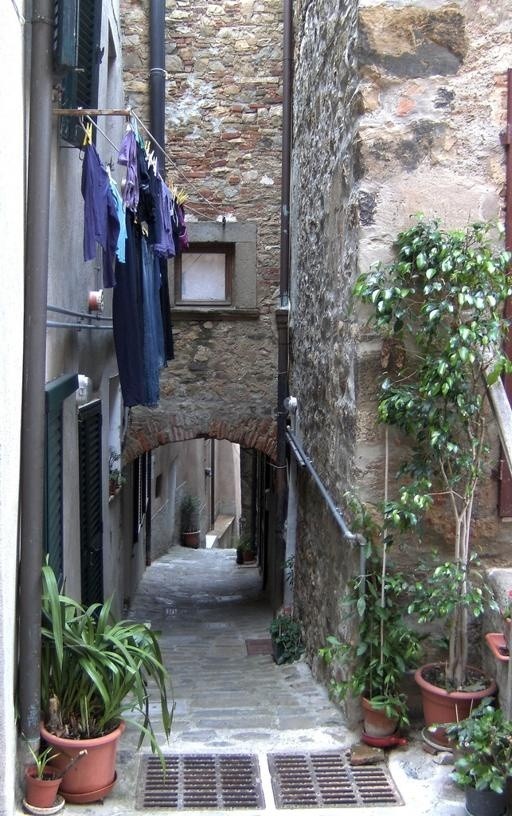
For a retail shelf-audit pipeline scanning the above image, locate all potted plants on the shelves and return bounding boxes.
[266,613,306,665]
[233,534,255,561]
[353,209,511,757]
[180,494,200,548]
[109,451,129,495]
[21,731,64,808]
[319,486,426,738]
[434,698,511,816]
[38,553,176,805]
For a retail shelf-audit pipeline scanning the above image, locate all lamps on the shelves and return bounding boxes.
[283,396,297,410]
